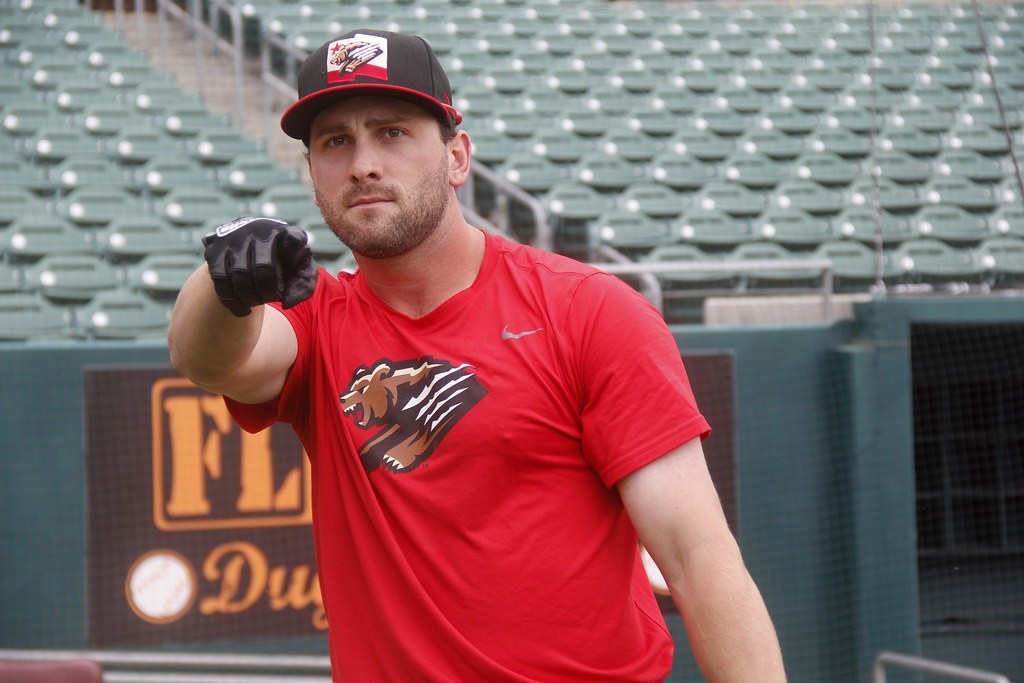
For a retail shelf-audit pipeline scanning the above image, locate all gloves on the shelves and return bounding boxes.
[200,217,318,318]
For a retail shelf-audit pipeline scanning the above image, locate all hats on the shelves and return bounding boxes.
[281,29,463,140]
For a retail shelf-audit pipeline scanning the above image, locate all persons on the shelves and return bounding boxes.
[166,30,790,683]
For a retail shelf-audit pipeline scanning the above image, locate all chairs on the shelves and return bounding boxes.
[0,0,1024,347]
